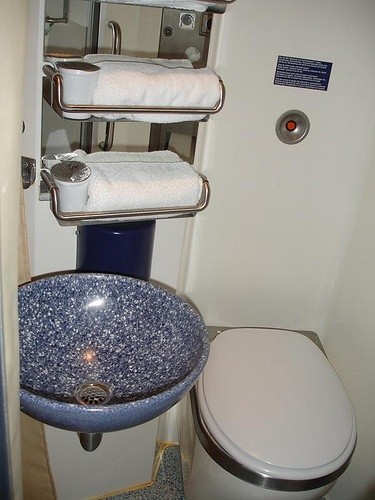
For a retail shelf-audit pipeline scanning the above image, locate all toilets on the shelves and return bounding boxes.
[179,325,358,500]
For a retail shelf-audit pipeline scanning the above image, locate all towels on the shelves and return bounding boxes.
[54,147,203,224]
[82,53,222,125]
[95,0,209,14]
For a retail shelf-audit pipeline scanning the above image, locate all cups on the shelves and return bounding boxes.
[41,152,79,170]
[50,160,92,212]
[54,60,101,121]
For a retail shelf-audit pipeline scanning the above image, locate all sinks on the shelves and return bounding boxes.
[19,272,210,434]
